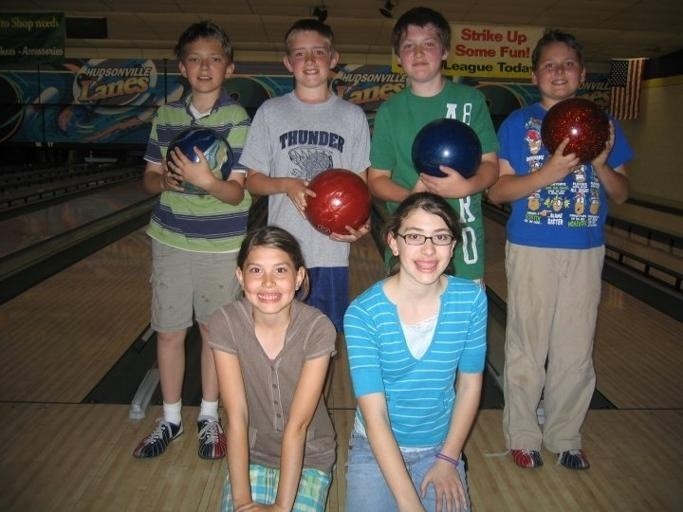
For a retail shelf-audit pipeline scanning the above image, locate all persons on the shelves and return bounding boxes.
[131,20,252,459]
[342,192,487,511]
[238,20,371,405]
[206,226,337,512]
[483,31,633,469]
[366,5,499,292]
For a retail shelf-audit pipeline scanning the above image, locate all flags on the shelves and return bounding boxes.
[607,57,644,121]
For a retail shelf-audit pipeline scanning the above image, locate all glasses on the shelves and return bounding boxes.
[396,232,456,246]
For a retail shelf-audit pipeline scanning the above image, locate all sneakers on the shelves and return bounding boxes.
[133,418,184,458]
[554,449,589,471]
[510,448,544,468]
[197,416,227,459]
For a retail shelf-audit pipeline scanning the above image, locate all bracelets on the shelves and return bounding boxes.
[435,453,459,466]
